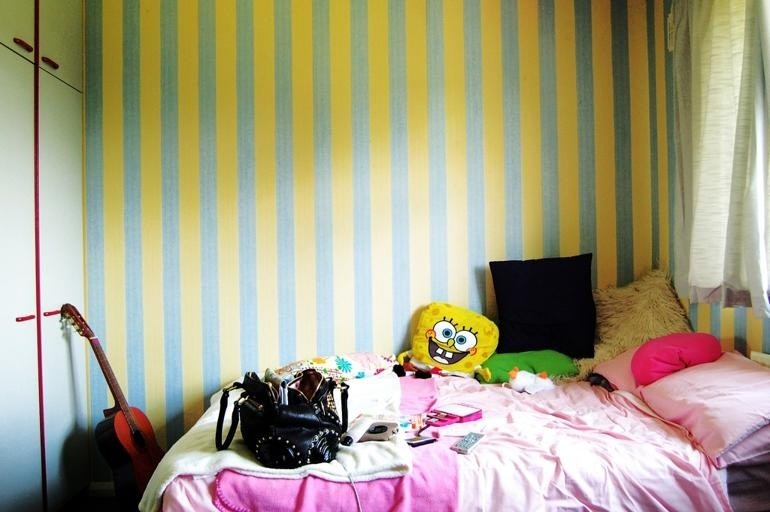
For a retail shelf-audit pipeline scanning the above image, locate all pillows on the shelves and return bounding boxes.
[585,343,769,472]
[480,346,578,383]
[487,253,601,360]
[631,330,724,388]
[560,253,696,382]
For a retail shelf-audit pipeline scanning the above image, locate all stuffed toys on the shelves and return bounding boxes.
[507,368,555,395]
[392,302,500,384]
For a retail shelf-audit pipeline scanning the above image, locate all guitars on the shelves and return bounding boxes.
[61,304,165,511]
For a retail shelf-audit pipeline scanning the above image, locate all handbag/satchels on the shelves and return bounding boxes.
[215,366,351,468]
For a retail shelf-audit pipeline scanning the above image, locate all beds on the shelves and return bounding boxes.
[138,349,770,510]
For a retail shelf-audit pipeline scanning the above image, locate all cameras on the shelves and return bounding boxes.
[405,436,436,447]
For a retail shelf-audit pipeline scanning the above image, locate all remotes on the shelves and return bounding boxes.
[450,431,487,454]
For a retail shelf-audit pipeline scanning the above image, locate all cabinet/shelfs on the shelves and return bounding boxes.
[0,1,88,511]
